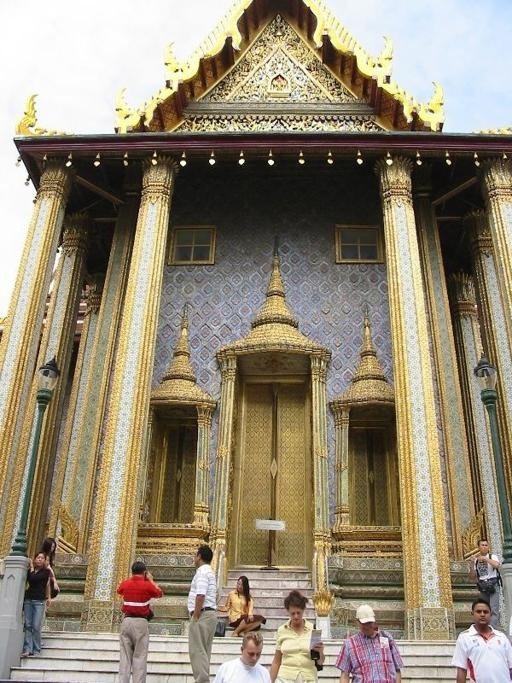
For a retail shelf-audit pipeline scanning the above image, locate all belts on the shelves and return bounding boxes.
[191,607,214,615]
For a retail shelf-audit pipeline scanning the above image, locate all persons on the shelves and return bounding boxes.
[20,537,60,656]
[452,597,512,683]
[213,630,273,683]
[117,562,163,683]
[186,545,218,683]
[270,590,325,683]
[217,575,266,637]
[335,604,403,683]
[474,540,500,630]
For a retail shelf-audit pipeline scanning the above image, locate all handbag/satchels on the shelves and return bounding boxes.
[476,576,499,593]
[215,621,225,637]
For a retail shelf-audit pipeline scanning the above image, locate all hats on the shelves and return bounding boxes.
[356,604,376,624]
